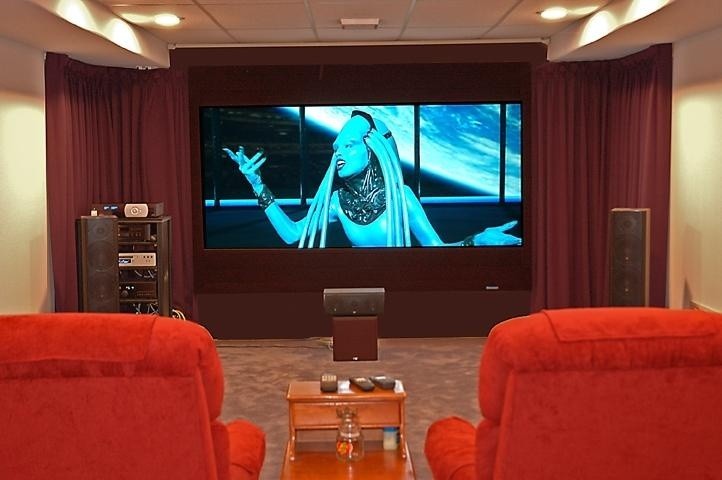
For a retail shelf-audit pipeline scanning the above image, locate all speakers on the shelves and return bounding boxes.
[639,269,644,273]
[77,215,120,312]
[609,207,651,313]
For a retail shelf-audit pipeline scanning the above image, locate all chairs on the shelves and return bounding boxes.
[0,313,265,479]
[424,307,722,480]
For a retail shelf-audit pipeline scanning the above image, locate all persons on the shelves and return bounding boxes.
[223,101,522,247]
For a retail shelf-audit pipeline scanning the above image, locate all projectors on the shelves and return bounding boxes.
[322,288,387,317]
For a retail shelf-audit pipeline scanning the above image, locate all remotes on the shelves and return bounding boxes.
[320,371,339,392]
[350,374,375,391]
[372,375,395,391]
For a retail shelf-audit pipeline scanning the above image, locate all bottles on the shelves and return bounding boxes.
[335,412,365,463]
[383,428,400,450]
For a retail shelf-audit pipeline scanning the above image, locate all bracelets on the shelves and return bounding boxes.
[460,230,474,247]
[256,184,276,209]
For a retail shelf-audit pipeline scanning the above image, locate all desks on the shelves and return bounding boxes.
[280,377,415,480]
